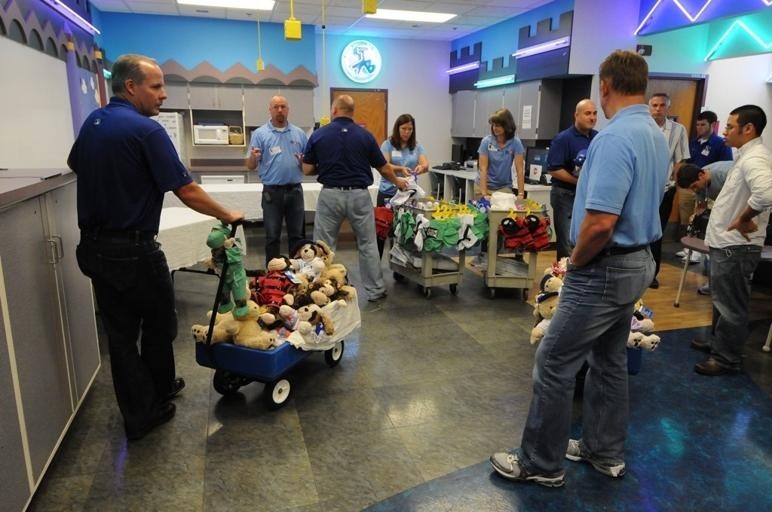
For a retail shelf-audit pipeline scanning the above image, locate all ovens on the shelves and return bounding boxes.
[200,174,245,184]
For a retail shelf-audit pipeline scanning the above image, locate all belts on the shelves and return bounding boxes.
[707,246,763,254]
[321,183,367,190]
[263,181,303,190]
[82,226,157,243]
[598,242,655,259]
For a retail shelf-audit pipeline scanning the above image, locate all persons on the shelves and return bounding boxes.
[546,99,599,265]
[681,110,732,172]
[487,47,671,489]
[373,111,430,259]
[243,93,314,264]
[688,103,771,375]
[300,93,409,305]
[473,107,525,260]
[68,55,245,444]
[671,161,733,214]
[646,92,691,290]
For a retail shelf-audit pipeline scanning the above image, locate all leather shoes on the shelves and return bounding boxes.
[694,356,746,376]
[123,402,184,444]
[142,377,189,403]
[689,338,714,355]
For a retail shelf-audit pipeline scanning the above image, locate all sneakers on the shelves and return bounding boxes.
[367,289,390,302]
[489,449,567,491]
[563,437,629,480]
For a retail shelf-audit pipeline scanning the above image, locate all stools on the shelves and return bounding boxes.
[760,245,771,352]
[673,236,710,308]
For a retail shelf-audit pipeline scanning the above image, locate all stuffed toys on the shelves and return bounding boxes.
[186,218,360,361]
[525,249,663,361]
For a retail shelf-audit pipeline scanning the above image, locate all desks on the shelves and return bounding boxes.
[429,163,553,210]
[154,205,243,319]
[162,182,382,239]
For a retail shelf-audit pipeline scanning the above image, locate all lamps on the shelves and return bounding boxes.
[283,0,301,41]
[255,9,265,74]
[361,0,377,15]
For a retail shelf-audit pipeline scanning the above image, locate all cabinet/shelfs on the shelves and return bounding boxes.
[0,179,101,511]
[450,79,562,141]
[106,78,314,183]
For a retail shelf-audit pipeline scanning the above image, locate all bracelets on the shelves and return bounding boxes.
[518,192,524,196]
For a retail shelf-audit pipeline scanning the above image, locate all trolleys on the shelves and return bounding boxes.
[387,197,476,298]
[193,217,360,408]
[481,190,551,302]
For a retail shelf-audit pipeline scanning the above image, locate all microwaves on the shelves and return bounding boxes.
[194,122,229,145]
